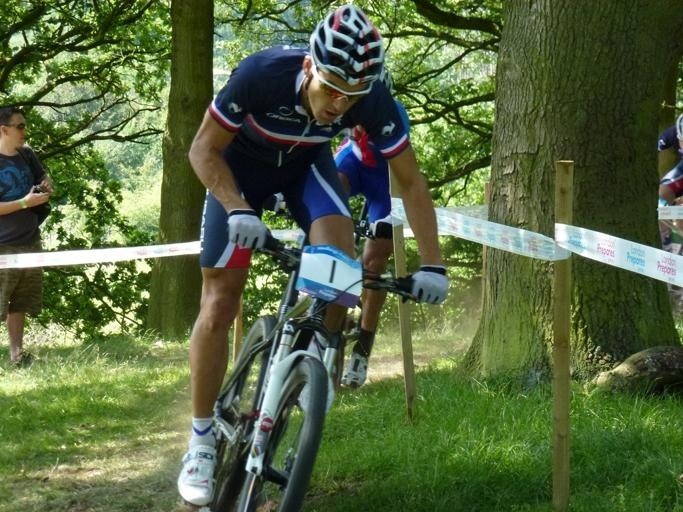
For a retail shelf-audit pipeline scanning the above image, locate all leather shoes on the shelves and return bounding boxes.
[227,208,267,250]
[411,264,449,305]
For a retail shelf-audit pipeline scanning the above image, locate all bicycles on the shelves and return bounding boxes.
[211,196,420,512]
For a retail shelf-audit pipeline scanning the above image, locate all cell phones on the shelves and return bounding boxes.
[30,188,50,214]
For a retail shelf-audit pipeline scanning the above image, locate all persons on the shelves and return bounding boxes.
[317,70,412,388]
[0,105,55,368]
[172,4,453,508]
[656,111,683,252]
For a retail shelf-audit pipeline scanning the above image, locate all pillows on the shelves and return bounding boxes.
[309,3,385,86]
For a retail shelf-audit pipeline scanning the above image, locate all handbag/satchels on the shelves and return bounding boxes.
[18,199,27,210]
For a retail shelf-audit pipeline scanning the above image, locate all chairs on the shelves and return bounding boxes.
[10,350,40,369]
[177,444,218,506]
[346,351,368,389]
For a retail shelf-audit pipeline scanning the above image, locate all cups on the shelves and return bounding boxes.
[309,66,373,101]
[4,123,26,131]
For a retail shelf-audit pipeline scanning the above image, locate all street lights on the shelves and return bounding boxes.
[35,177,51,227]
